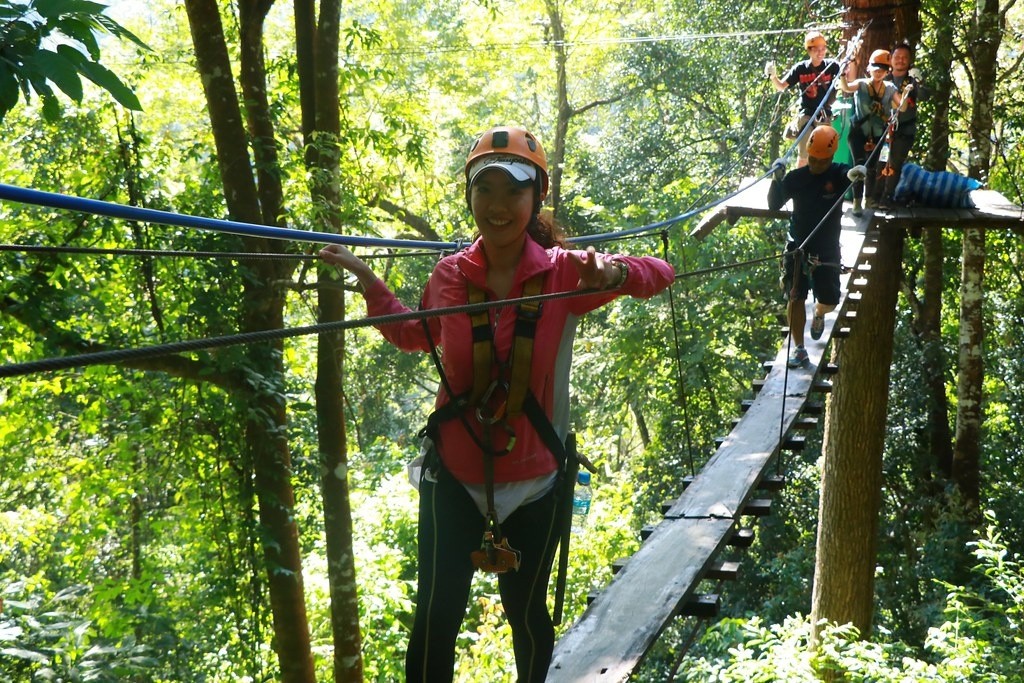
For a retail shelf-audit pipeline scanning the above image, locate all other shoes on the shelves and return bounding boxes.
[852,207,862,215]
[878,202,889,210]
[871,200,878,208]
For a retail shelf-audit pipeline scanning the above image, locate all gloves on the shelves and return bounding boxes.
[772,157,788,179]
[846,165,867,183]
[765,62,776,79]
[908,68,923,82]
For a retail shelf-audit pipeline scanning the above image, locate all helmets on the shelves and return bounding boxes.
[464,126,548,201]
[869,50,891,65]
[805,32,830,50]
[806,125,838,159]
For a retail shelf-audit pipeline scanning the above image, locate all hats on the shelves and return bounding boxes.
[468,154,536,192]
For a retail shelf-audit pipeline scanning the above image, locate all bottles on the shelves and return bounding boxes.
[570,471,593,532]
[878,143,889,162]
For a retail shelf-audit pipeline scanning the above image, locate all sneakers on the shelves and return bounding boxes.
[788,344,808,367]
[810,304,824,340]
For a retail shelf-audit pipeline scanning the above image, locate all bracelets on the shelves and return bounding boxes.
[608,262,628,290]
[841,73,845,76]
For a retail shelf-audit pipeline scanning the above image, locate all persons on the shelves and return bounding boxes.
[841,44,918,217]
[317,127,676,683]
[766,32,862,169]
[767,125,867,368]
